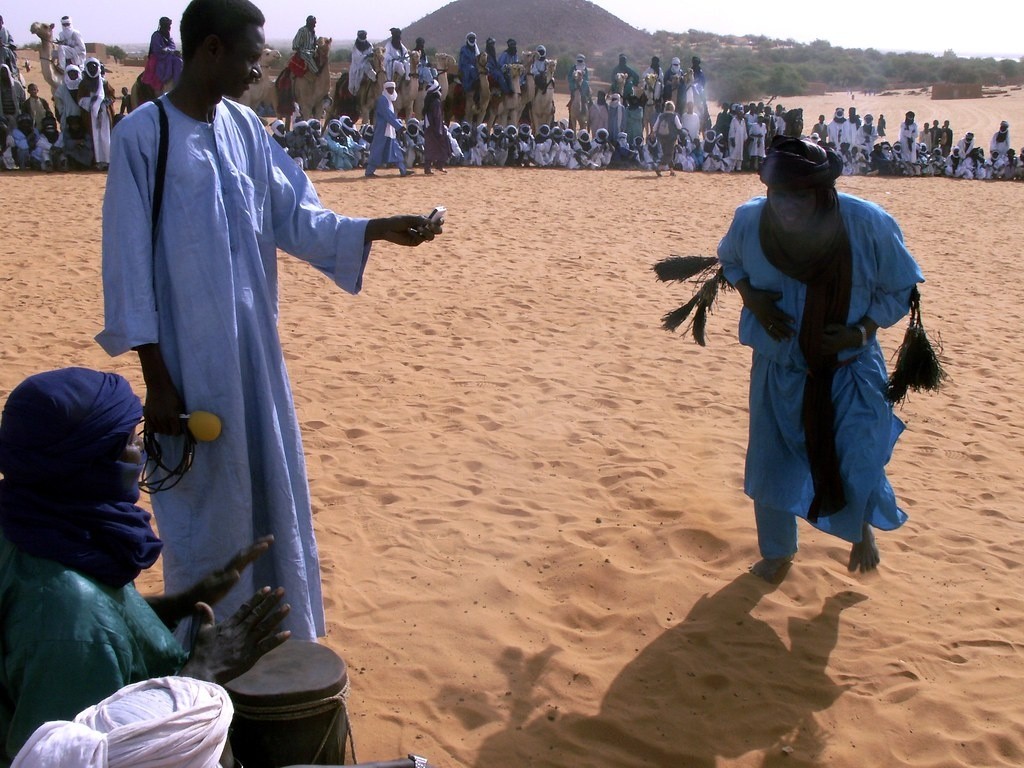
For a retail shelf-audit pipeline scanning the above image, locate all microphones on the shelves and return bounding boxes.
[179,409,222,440]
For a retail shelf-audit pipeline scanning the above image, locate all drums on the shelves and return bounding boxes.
[223,637,352,768]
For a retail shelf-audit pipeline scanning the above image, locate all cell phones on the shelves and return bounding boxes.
[422,206,447,228]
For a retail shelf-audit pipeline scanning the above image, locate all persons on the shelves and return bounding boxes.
[98,1,446,660]
[0,17,1023,187]
[0,363,295,768]
[716,138,926,585]
